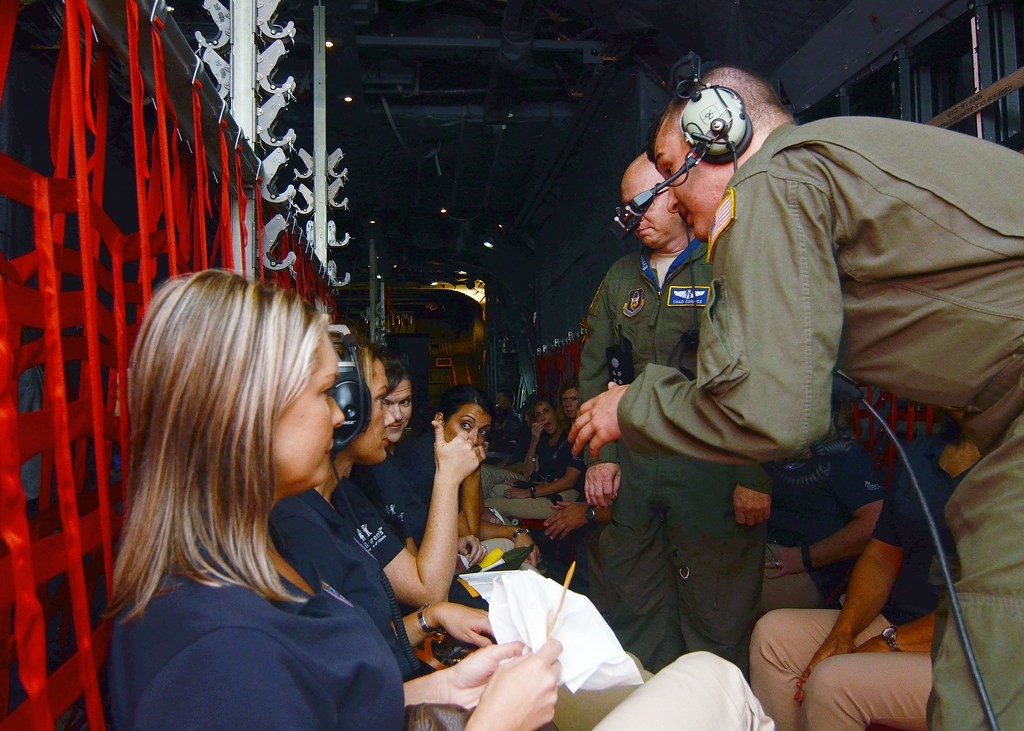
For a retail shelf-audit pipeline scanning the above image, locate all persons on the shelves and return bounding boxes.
[269,337,982,731]
[580,150,782,683]
[103,270,773,731]
[569,66,1024,731]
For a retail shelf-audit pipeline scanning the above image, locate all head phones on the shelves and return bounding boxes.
[324,323,372,457]
[670,51,753,163]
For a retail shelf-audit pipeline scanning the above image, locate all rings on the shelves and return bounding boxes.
[482,546,484,548]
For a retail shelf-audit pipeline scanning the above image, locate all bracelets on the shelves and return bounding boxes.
[489,507,495,513]
[417,604,441,632]
[801,543,813,570]
[529,486,536,498]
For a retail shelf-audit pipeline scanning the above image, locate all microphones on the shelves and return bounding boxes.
[610,109,735,240]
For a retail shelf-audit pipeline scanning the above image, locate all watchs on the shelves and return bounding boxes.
[883,626,899,651]
[531,457,536,462]
[512,528,532,541]
[585,504,598,524]
